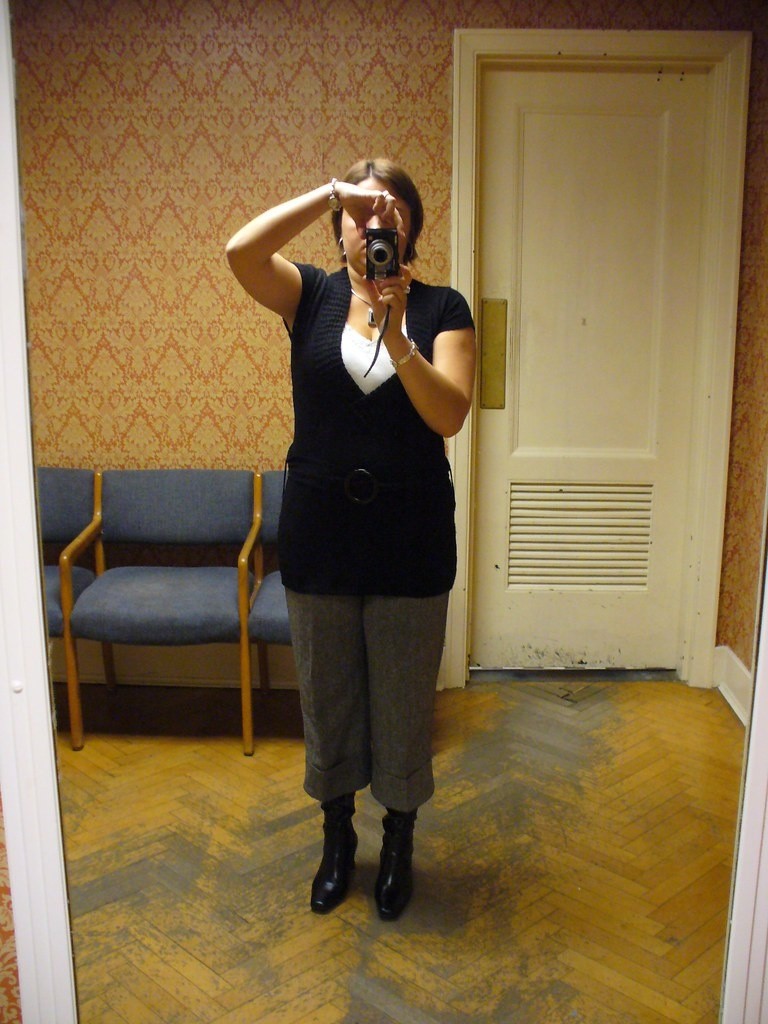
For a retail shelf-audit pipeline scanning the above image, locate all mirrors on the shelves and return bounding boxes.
[0,0,768,1024]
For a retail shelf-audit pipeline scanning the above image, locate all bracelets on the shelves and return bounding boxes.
[390,341,418,368]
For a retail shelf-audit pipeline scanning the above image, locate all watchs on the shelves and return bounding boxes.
[328,178,342,211]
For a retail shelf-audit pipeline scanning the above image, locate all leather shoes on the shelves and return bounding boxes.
[310,820,357,914]
[375,814,414,920]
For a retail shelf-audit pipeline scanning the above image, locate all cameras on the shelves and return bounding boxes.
[364,228,399,280]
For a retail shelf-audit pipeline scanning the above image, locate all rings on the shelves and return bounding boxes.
[383,190,389,197]
[404,285,410,294]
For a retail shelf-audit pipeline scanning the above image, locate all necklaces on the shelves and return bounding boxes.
[352,289,376,324]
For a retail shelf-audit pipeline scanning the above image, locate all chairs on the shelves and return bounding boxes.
[36,466,294,756]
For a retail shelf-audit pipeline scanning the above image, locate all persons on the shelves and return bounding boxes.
[225,158,475,922]
